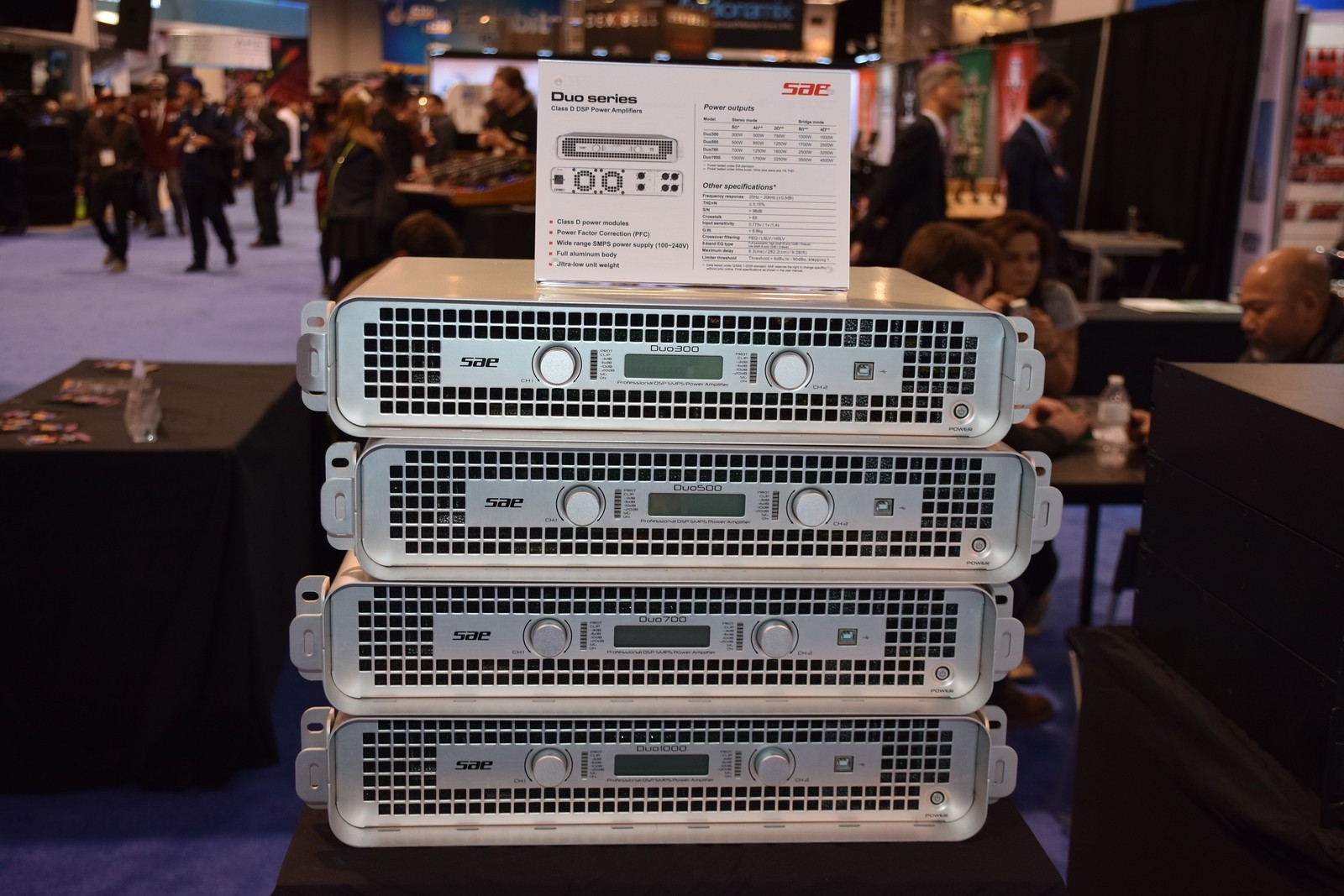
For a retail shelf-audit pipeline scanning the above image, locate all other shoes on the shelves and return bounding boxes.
[109,259,126,271]
[227,249,238,265]
[104,253,116,264]
[185,261,207,271]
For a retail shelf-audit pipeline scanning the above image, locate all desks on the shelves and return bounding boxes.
[0,356,338,800]
[1072,298,1252,408]
[1058,226,1185,304]
[1036,394,1153,626]
[1067,628,1344,896]
[265,794,1069,896]
[396,155,537,259]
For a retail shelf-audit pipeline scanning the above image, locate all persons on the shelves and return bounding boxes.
[850,61,1344,719]
[0,60,538,299]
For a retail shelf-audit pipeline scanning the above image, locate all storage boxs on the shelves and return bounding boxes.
[1131,357,1344,825]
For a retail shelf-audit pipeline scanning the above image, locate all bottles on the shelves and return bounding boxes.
[1094,375,1131,469]
[1127,208,1139,234]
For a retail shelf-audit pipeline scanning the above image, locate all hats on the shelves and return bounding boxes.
[143,73,169,87]
[181,76,207,99]
